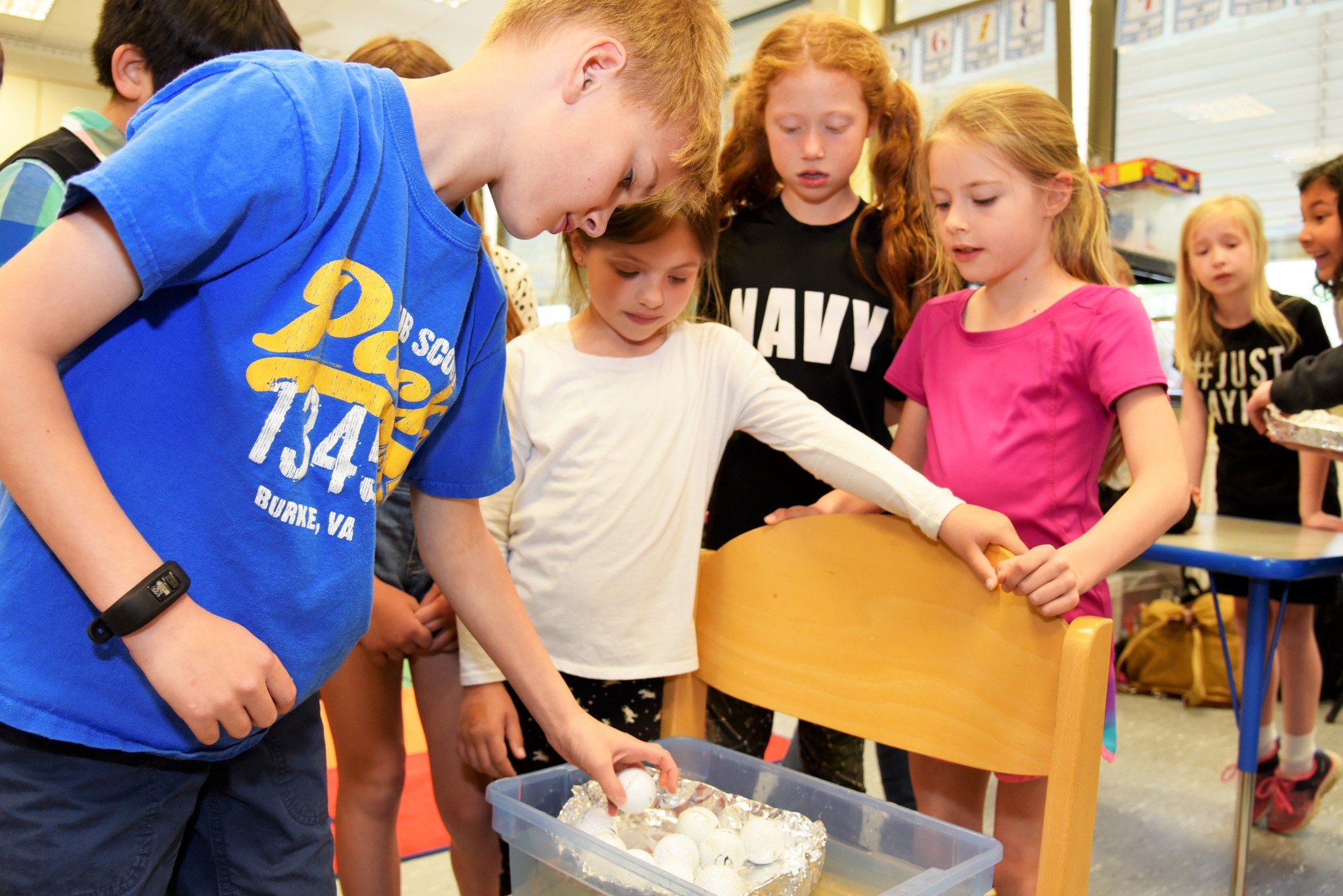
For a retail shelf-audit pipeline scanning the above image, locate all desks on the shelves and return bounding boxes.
[1135,514,1343,896]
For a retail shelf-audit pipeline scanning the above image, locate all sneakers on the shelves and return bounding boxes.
[1221,733,1282,826]
[1254,747,1343,834]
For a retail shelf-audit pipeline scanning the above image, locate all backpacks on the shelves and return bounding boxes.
[1114,593,1247,707]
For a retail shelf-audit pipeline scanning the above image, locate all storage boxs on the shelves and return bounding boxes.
[485,736,1003,896]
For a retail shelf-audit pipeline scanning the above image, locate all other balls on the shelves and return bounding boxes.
[614,767,655,817]
[575,806,792,896]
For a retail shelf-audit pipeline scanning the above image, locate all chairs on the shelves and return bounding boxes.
[506,506,1113,896]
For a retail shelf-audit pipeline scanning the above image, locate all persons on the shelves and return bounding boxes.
[453,190,1029,896]
[709,7,940,840]
[766,80,1188,896]
[1176,156,1343,836]
[316,36,541,896]
[0,0,728,896]
[0,0,301,270]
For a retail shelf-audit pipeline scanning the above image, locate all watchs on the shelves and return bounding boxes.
[87,559,191,644]
